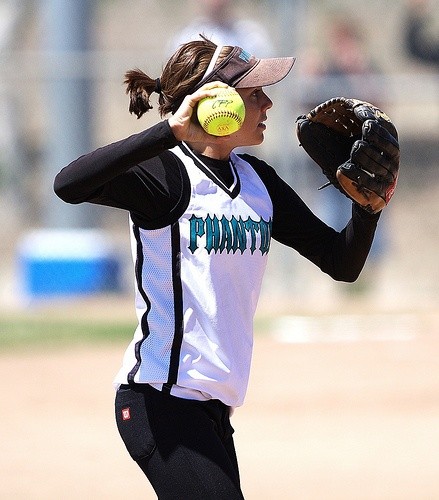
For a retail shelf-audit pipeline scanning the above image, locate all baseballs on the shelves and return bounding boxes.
[198,86,247,137]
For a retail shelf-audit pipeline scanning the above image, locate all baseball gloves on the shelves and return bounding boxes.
[297,96,400,213]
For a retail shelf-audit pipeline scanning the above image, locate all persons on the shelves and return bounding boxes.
[167,0,394,265]
[54,36,402,500]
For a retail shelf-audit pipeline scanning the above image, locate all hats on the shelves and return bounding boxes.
[193,46,296,91]
